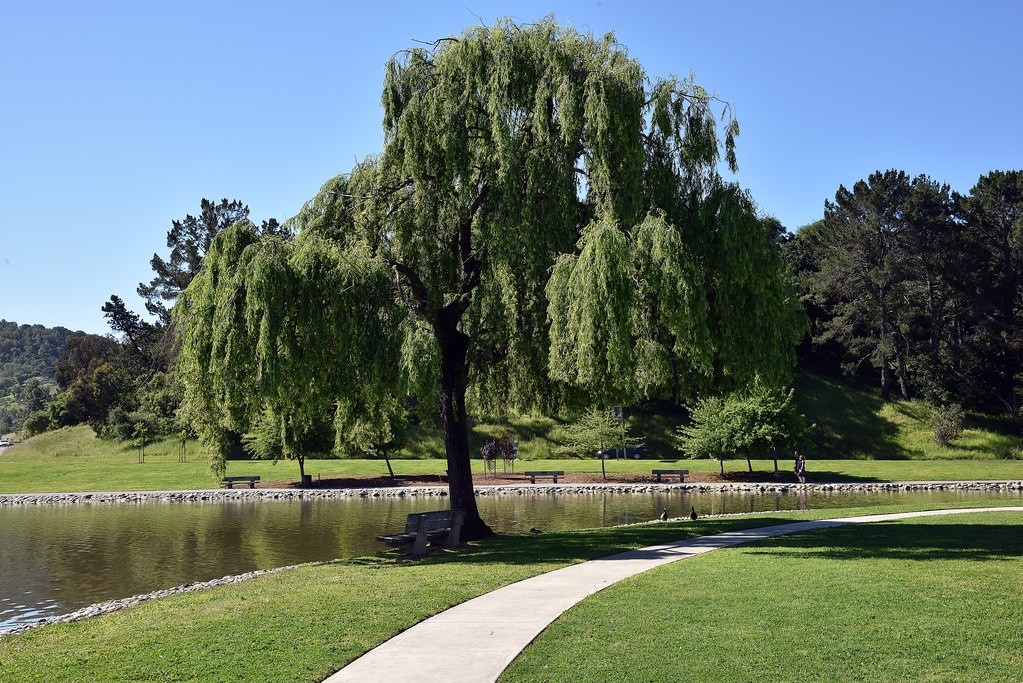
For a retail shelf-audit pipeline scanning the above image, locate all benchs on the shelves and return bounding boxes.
[524,471,564,485]
[220,476,260,489]
[652,469,690,483]
[375,508,466,559]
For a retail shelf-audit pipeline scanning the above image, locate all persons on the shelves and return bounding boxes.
[794,451,801,475]
[797,455,806,483]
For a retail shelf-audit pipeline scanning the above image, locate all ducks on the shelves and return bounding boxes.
[660,507,667,521]
[690,506,697,519]
